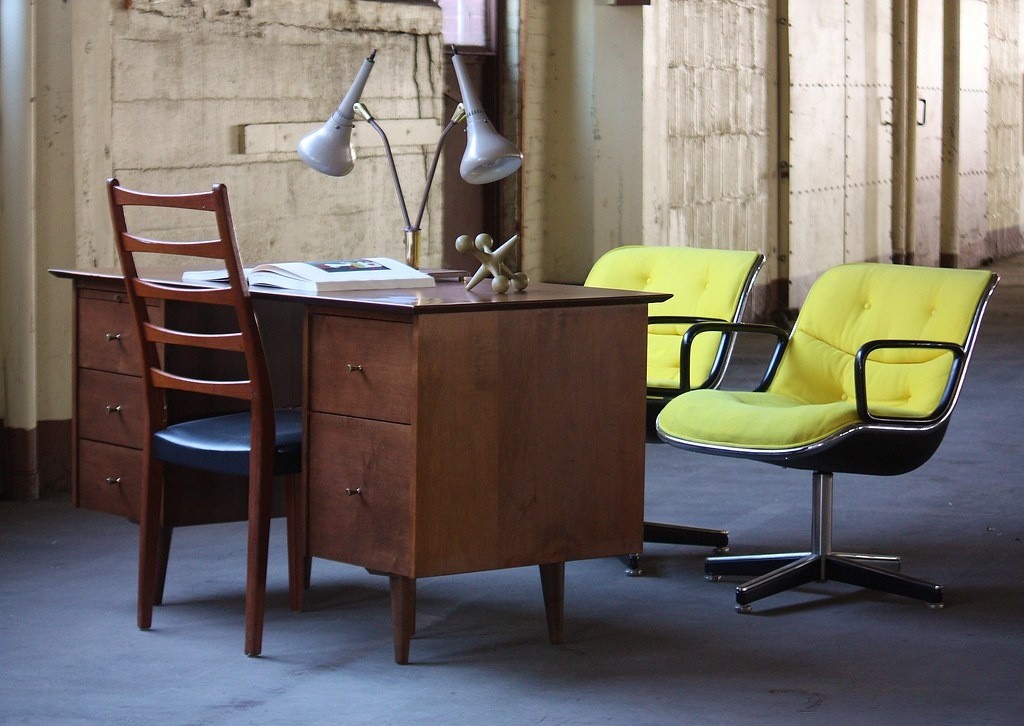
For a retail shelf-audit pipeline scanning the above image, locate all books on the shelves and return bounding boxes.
[182,257,436,293]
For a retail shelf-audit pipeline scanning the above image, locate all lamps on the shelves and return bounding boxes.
[296,42,524,283]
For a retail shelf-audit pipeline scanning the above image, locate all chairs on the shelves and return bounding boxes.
[654,262,1001,617]
[105,177,417,658]
[582,243,766,577]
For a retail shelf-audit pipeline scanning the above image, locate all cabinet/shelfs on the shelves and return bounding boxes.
[46,267,674,666]
